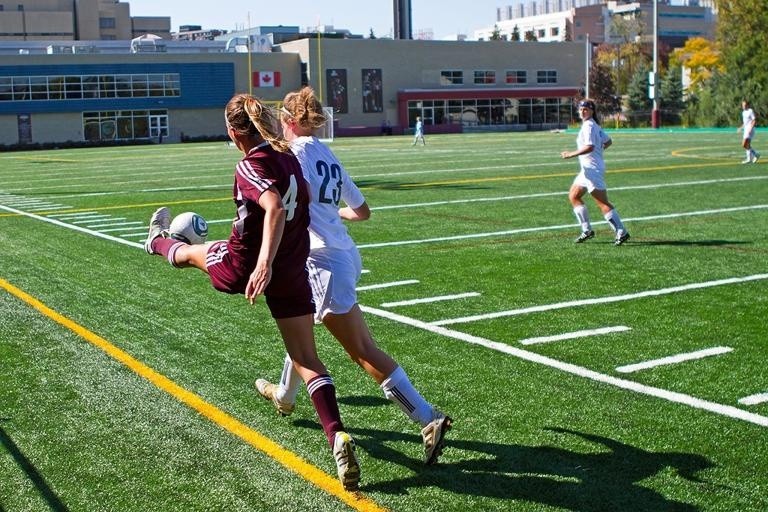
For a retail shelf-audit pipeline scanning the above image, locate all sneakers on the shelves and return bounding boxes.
[333,431,361,492]
[146,207,171,256]
[752,154,760,163]
[420,406,454,468]
[742,161,750,164]
[573,230,595,244]
[614,232,630,247]
[256,378,296,415]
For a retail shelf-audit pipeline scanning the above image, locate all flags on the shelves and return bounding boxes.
[252,71,280,87]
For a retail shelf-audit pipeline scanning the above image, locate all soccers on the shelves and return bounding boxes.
[170,212,208,245]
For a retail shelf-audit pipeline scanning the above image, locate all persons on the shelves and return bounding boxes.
[559,99,629,246]
[411,116,425,146]
[146,93,361,491]
[737,99,760,164]
[255,86,454,466]
